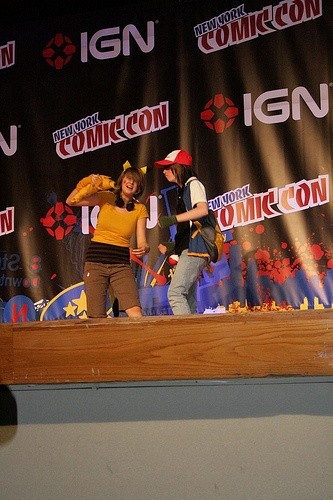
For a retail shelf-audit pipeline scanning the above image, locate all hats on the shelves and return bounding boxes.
[154,149,192,169]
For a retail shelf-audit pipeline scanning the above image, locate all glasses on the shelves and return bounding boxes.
[163,166,170,170]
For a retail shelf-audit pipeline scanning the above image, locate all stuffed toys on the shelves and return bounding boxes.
[71,174,119,205]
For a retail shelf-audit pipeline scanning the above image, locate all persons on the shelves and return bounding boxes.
[158,149,224,315]
[65,165,151,318]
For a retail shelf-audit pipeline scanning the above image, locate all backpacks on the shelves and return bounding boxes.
[183,179,224,263]
